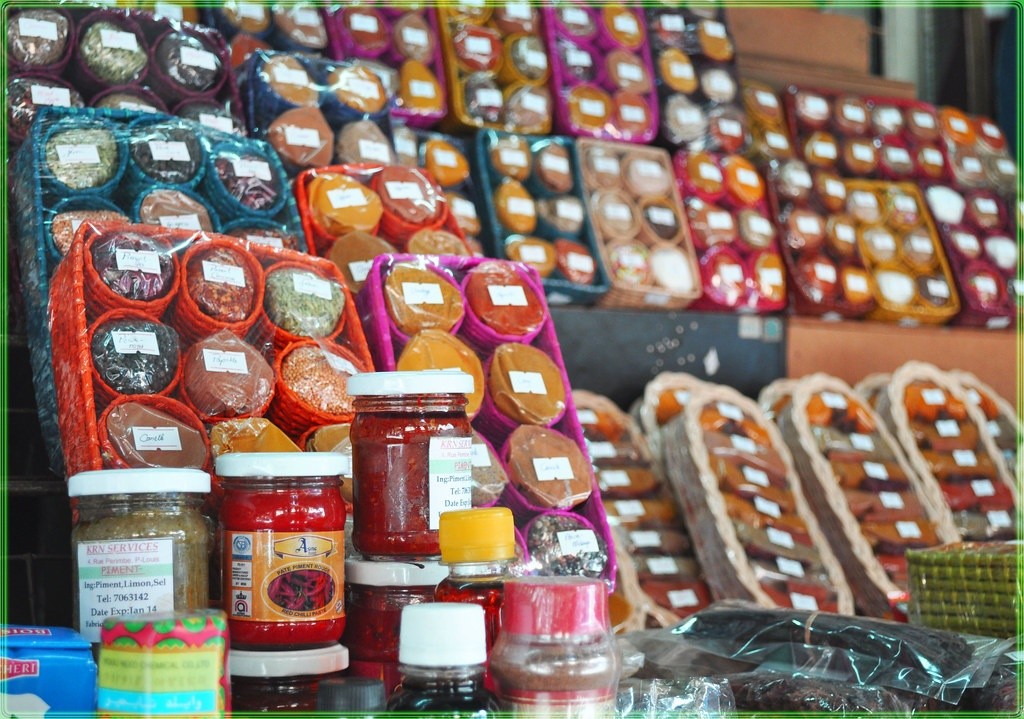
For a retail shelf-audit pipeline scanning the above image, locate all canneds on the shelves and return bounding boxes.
[67,366,475,712]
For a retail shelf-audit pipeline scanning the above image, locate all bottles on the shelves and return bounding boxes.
[488,581,622,719]
[386,603,501,719]
[433,507,525,699]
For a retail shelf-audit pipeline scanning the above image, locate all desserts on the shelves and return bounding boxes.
[7,3,1019,620]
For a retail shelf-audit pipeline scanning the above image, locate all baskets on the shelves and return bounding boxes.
[5,9,1024,631]
